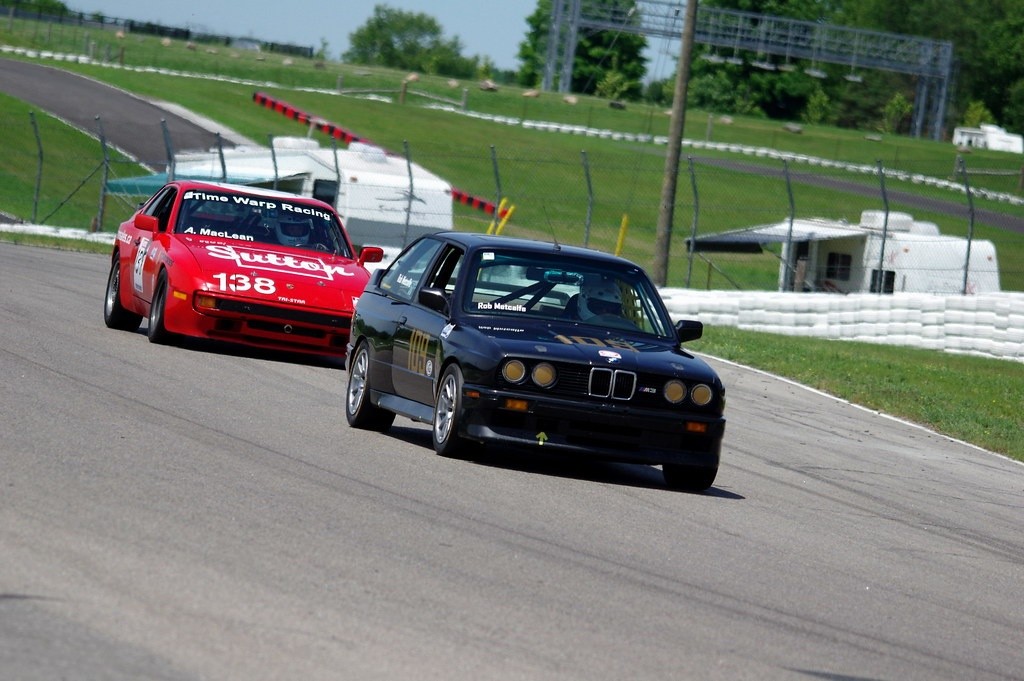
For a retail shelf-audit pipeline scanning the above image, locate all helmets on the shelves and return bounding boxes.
[274,211,312,246]
[577,277,624,322]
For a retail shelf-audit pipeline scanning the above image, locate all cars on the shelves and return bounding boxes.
[103,180,384,357]
[343,230,727,492]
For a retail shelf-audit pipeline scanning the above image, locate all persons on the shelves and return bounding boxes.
[574,279,623,320]
[275,211,310,246]
